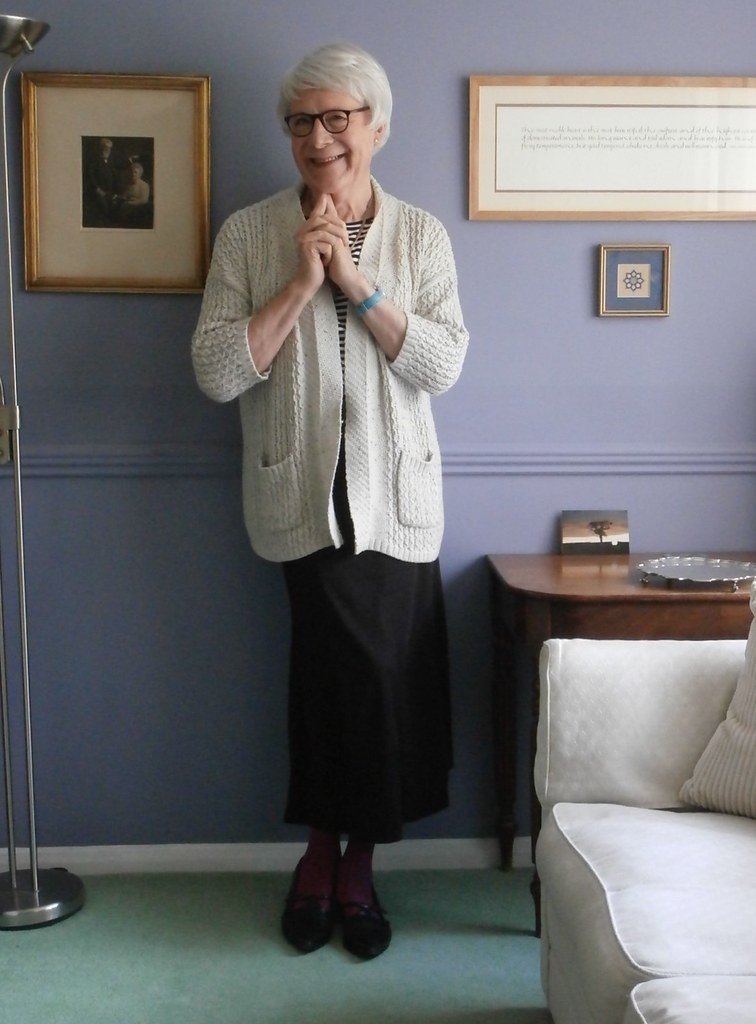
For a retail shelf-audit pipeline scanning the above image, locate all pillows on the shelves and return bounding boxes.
[677,579,755,819]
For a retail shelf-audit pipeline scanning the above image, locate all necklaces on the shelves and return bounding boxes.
[309,195,373,252]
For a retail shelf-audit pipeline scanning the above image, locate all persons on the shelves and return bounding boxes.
[89,138,149,217]
[193,42,468,956]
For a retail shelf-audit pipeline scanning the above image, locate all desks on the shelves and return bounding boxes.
[485,553,755,937]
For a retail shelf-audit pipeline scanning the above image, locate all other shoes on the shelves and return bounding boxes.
[281,853,335,954]
[337,868,392,960]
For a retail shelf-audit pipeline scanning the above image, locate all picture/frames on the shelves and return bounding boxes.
[19,72,213,294]
[598,244,670,317]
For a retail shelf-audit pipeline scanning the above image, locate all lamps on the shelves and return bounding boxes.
[0,14,83,930]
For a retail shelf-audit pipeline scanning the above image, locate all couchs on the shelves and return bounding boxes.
[535,639,756,1024]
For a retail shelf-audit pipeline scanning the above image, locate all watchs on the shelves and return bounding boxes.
[354,286,386,312]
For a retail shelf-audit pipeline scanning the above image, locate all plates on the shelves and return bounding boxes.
[637,556,756,581]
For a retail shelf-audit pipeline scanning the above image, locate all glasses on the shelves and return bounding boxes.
[284,106,370,137]
